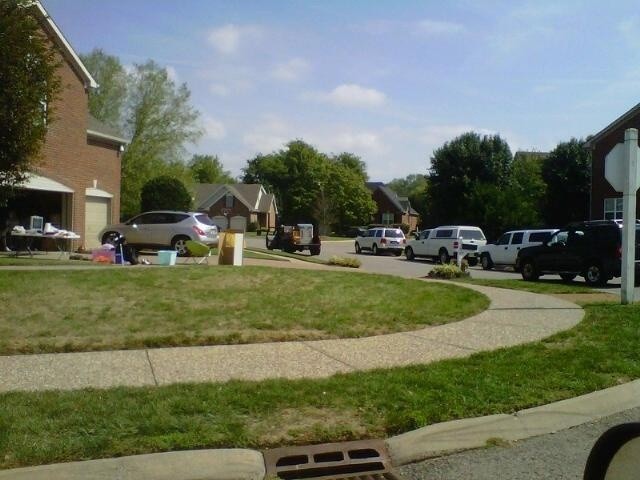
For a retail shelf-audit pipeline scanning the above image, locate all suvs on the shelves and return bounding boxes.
[98,210,219,257]
[355,219,640,287]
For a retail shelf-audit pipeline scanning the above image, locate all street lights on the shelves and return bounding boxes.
[317,182,326,235]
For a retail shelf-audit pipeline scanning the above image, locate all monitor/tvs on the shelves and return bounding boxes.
[29,215,44,232]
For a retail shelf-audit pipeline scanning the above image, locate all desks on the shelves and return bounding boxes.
[11,231,81,257]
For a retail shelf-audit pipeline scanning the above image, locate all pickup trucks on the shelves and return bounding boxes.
[266,222,321,254]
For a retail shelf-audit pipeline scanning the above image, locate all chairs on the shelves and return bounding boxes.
[182,239,210,265]
